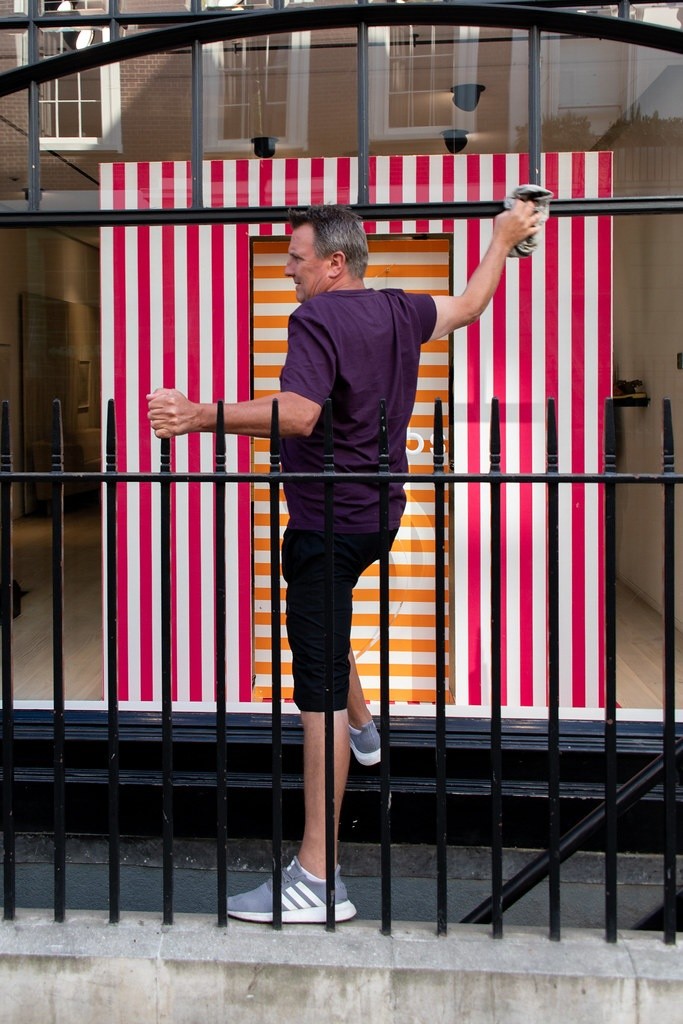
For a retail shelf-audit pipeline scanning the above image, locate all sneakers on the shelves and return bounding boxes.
[349,721,381,766]
[227,856,356,923]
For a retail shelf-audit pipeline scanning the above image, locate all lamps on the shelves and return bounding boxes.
[251,137,279,159]
[451,84,486,111]
[45,0,95,51]
[442,129,468,155]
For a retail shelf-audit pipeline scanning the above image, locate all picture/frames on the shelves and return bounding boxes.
[78,361,92,408]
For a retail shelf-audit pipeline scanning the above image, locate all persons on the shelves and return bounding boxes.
[148,198,544,923]
